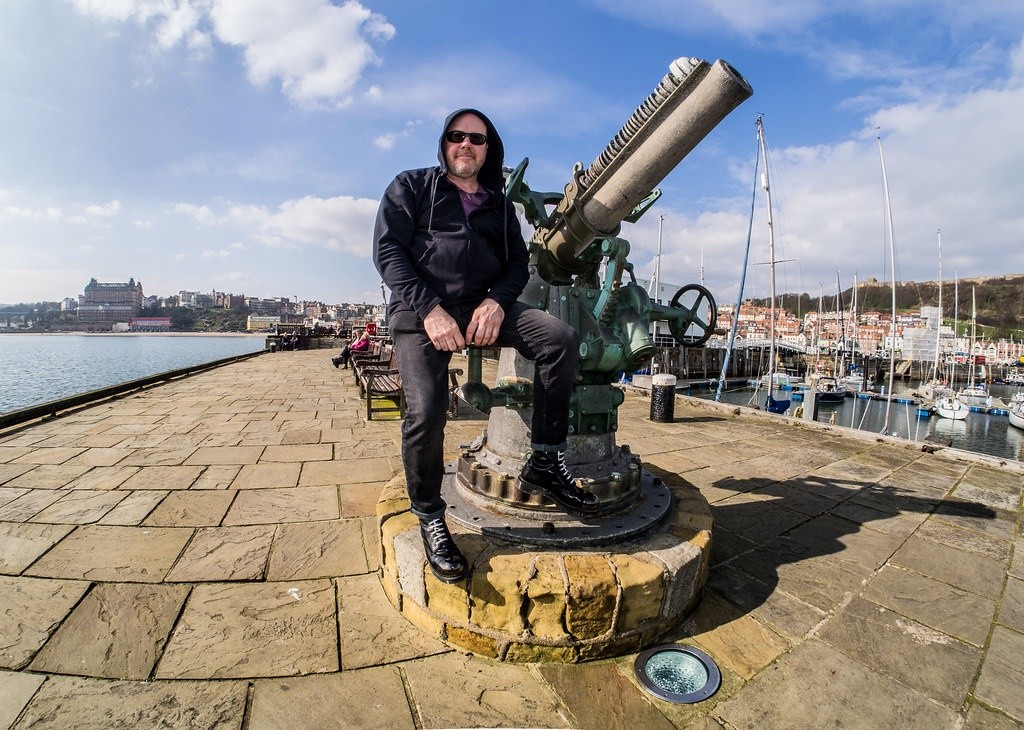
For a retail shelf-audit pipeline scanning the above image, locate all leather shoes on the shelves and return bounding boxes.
[514,449,601,519]
[419,515,470,584]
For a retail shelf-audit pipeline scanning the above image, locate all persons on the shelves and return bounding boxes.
[307,322,348,340]
[373,108,601,583]
[279,331,298,351]
[332,329,369,370]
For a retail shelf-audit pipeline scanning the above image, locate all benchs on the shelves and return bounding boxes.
[354,345,393,387]
[359,345,405,420]
[350,342,382,377]
[446,368,463,418]
[350,343,375,371]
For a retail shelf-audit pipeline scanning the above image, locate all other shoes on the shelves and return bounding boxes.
[342,366,348,369]
[337,358,343,364]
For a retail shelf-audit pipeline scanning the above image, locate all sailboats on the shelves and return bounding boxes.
[639,115,1024,438]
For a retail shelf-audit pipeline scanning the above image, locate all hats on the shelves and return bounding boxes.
[364,332,370,337]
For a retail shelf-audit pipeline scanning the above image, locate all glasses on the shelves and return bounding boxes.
[444,130,489,145]
